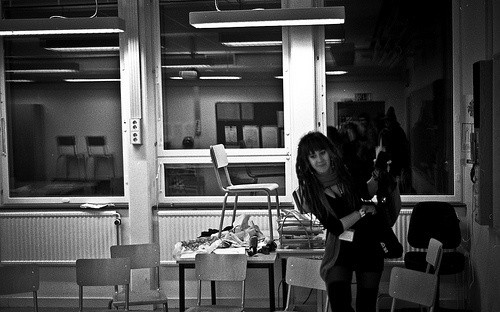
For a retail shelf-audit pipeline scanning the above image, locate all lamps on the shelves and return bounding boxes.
[0,0,126,37]
[189,0,345,29]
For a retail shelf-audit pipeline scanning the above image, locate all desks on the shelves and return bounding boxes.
[175,235,325,312]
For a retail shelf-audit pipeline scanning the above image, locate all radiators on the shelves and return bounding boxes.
[382,205,454,264]
[0,210,120,266]
[157,208,282,266]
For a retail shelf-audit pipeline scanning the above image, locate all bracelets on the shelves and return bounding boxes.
[359,208,365,218]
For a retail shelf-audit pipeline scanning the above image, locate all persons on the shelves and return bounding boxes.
[296,132,392,312]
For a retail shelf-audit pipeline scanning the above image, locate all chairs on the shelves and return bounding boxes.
[209,143,280,239]
[183,253,247,312]
[0,261,40,312]
[283,257,329,312]
[376,204,466,312]
[75,243,168,312]
[240,139,286,196]
[55,135,116,181]
